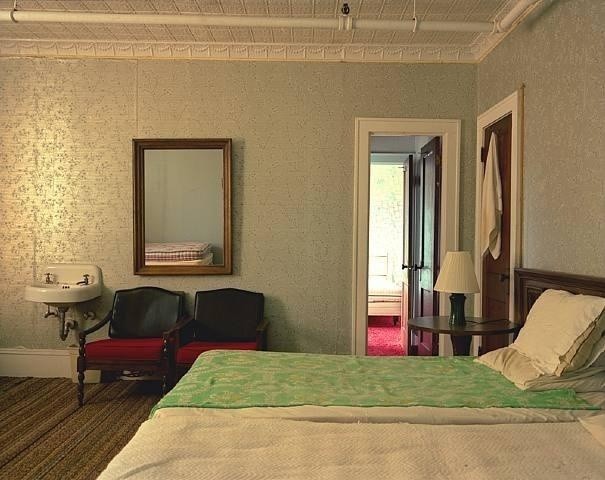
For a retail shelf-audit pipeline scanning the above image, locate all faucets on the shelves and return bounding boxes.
[76,274,89,285]
[44,273,52,284]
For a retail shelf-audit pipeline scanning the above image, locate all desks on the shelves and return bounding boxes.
[406,315,520,356]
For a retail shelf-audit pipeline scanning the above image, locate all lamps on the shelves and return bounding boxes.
[434,249,481,327]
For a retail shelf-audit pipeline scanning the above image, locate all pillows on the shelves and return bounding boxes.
[508,287,605,376]
[474,341,605,392]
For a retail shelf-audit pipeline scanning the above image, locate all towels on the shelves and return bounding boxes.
[478,130,503,260]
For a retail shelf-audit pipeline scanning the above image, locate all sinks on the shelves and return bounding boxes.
[24,283,100,308]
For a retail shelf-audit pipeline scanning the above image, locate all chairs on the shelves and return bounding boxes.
[77,286,189,407]
[174,288,270,381]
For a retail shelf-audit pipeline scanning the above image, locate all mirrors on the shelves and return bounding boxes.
[131,138,233,276]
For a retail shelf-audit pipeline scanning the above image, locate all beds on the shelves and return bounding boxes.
[145,242,214,265]
[368,250,401,327]
[94,349,605,480]
